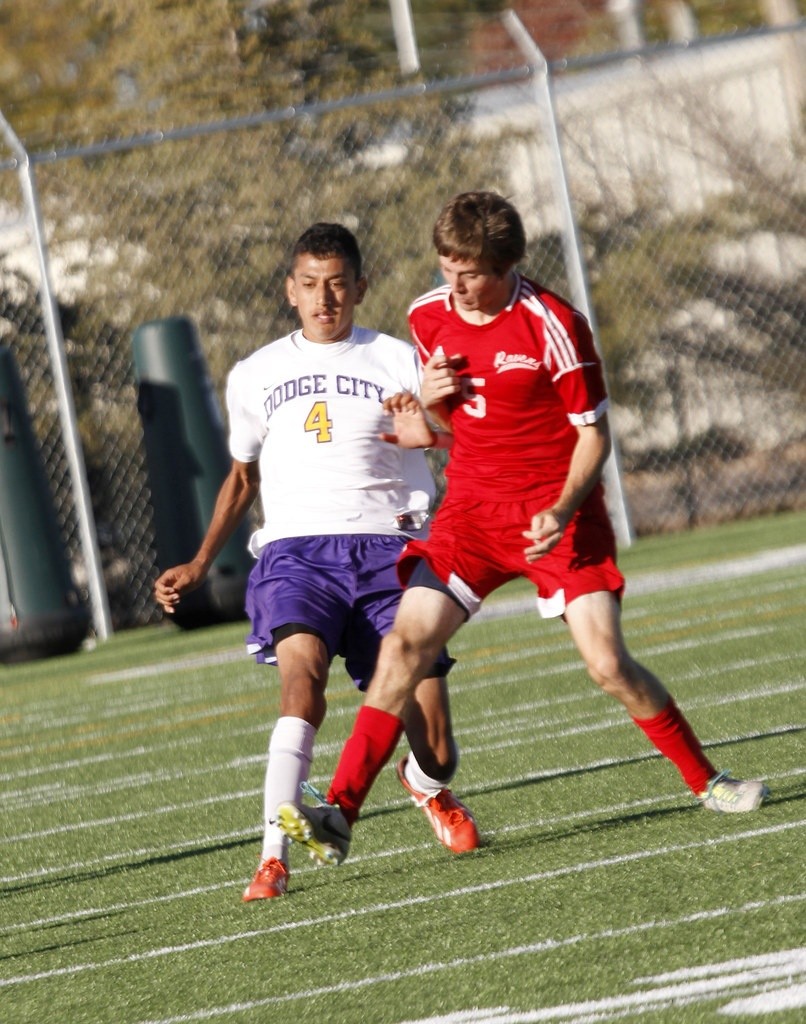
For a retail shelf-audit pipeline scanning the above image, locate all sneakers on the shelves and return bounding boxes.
[274,780,351,866]
[694,770,765,813]
[242,860,290,903]
[395,756,477,855]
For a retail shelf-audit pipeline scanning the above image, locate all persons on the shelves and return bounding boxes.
[276,190,765,867]
[153,221,479,902]
